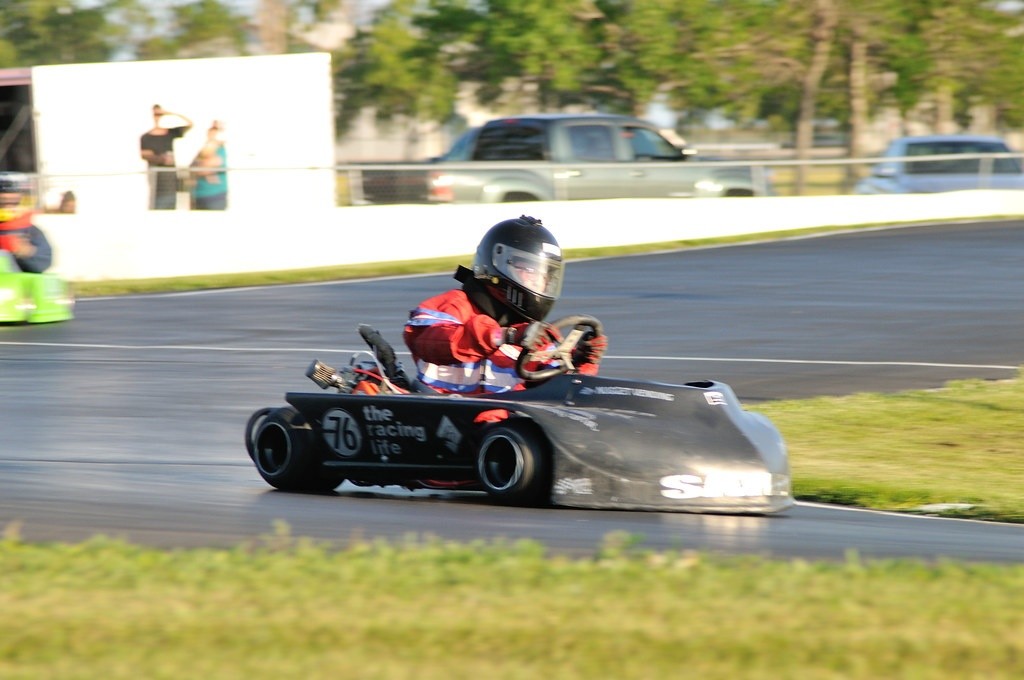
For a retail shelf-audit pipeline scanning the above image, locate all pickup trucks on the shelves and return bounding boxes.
[356,115,766,203]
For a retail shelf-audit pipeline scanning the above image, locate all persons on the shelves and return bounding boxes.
[402,218,609,394]
[140,105,194,209]
[1,175,51,272]
[189,123,227,210]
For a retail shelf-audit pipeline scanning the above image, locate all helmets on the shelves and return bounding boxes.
[452,215,564,321]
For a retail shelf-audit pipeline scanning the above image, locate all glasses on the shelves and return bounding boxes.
[514,266,554,290]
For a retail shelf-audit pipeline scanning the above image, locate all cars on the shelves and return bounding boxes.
[851,137,1024,195]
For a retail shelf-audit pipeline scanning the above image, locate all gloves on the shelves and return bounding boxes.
[574,333,609,364]
[505,321,564,353]
